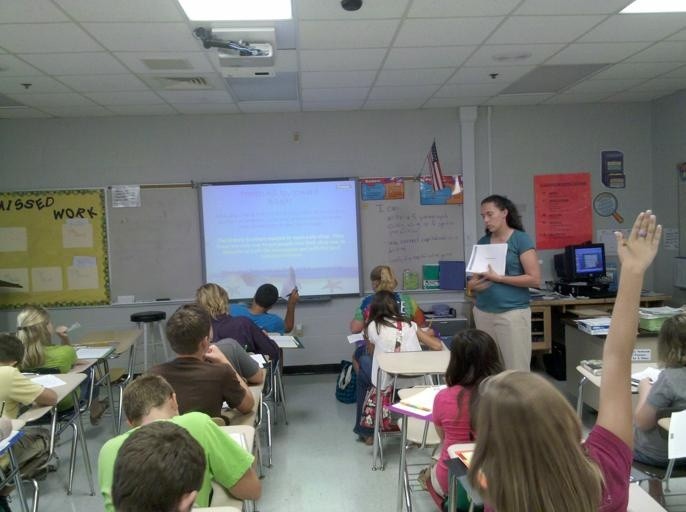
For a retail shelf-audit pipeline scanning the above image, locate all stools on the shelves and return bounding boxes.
[131,311,170,372]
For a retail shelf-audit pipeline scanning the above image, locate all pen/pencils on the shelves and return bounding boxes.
[623,238,627,247]
[429,316,433,329]
[286,290,298,298]
[64,322,82,334]
[1,402,5,417]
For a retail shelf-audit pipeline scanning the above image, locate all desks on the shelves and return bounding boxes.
[376,348,486,512]
[527,291,671,372]
[73,328,142,437]
[1,372,95,511]
[207,330,284,509]
[578,359,686,512]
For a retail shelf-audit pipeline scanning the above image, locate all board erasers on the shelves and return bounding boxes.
[156,298,170,301]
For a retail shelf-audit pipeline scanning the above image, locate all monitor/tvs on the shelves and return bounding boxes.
[554,243,606,282]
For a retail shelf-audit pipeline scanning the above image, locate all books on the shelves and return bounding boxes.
[422,264,439,289]
[260,335,299,347]
[630,366,662,396]
[571,317,616,336]
[466,242,508,277]
[385,383,449,420]
[579,359,602,377]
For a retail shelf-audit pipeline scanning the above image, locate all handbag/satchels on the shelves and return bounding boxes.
[357,382,408,432]
[336,360,357,404]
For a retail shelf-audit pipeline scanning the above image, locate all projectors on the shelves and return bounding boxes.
[217,43,277,78]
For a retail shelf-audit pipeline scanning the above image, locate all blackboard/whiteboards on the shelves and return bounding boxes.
[106,175,464,307]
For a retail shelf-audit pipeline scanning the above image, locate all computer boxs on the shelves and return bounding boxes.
[553,283,609,298]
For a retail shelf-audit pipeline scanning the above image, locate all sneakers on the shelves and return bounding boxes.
[91,396,111,426]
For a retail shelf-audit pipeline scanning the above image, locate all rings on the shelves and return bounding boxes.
[638,232,646,239]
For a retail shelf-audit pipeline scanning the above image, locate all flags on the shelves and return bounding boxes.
[426,141,445,192]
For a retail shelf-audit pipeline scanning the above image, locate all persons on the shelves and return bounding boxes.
[631,314,685,469]
[465,207,664,512]
[426,329,506,511]
[0,417,12,443]
[0,332,58,476]
[467,193,540,373]
[14,306,110,425]
[348,266,426,376]
[353,290,443,447]
